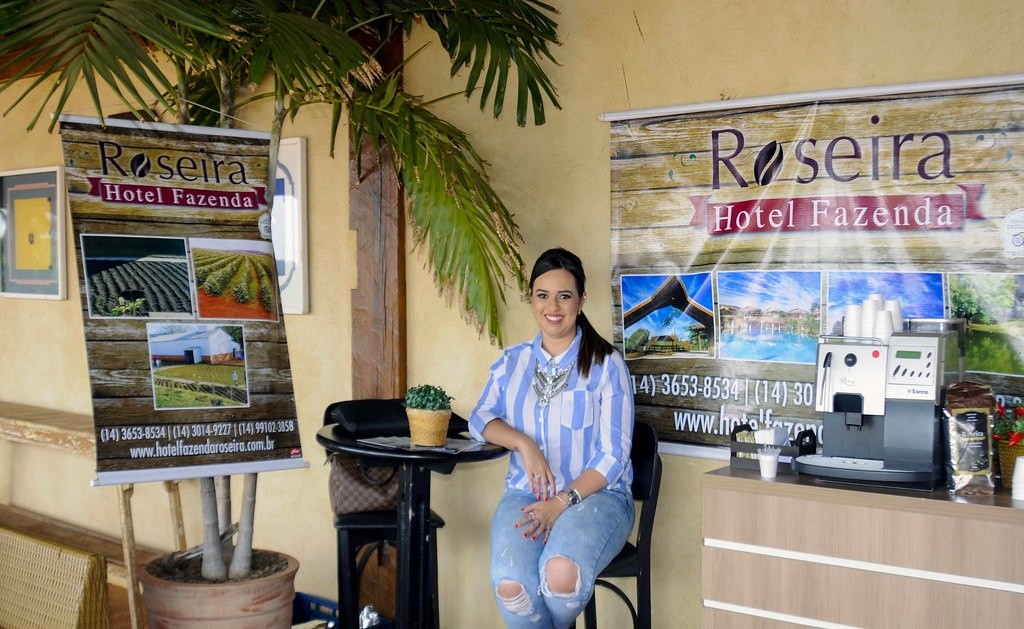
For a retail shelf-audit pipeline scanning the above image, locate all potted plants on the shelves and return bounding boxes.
[0,0,566,629]
[406,382,455,444]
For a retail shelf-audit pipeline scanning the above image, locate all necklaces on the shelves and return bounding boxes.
[533,359,576,407]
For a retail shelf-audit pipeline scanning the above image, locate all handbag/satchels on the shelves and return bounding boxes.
[323,451,401,517]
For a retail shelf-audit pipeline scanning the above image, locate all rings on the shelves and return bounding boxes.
[529,513,535,520]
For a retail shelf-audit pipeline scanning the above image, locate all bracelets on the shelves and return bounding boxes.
[555,496,568,509]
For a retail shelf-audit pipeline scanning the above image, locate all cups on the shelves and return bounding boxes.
[843,294,903,345]
[1011,456,1024,501]
[758,454,779,479]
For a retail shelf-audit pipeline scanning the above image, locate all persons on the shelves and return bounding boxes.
[468,246,636,629]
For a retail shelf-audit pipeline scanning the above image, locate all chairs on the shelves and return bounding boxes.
[565,416,663,629]
[323,397,445,629]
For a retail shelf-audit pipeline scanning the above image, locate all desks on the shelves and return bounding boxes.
[0,400,187,629]
[315,421,509,629]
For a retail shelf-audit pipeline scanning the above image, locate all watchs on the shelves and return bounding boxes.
[559,487,580,506]
